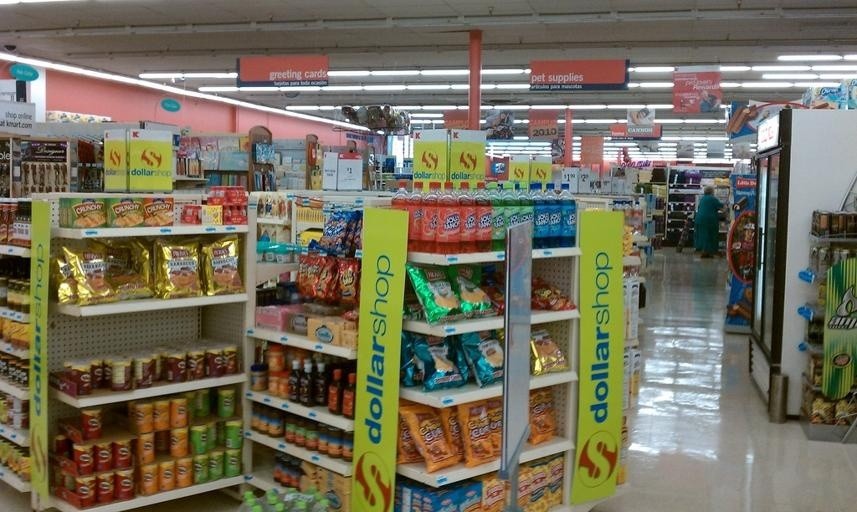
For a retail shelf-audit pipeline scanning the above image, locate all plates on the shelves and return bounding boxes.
[746,102,809,132]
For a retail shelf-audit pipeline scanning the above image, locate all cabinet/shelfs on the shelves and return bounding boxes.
[799,231,857,444]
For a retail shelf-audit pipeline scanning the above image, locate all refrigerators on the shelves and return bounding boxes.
[748,108,857,421]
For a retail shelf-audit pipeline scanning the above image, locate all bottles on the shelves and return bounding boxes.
[612,199,643,232]
[288,358,327,406]
[243,485,329,512]
[840,79,849,110]
[326,369,356,420]
[388,180,577,253]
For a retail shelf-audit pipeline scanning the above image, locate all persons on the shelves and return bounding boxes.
[629,108,648,126]
[700,89,715,111]
[494,112,512,130]
[694,184,728,258]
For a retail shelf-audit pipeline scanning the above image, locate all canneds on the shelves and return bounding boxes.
[250,402,353,462]
[0,275,31,483]
